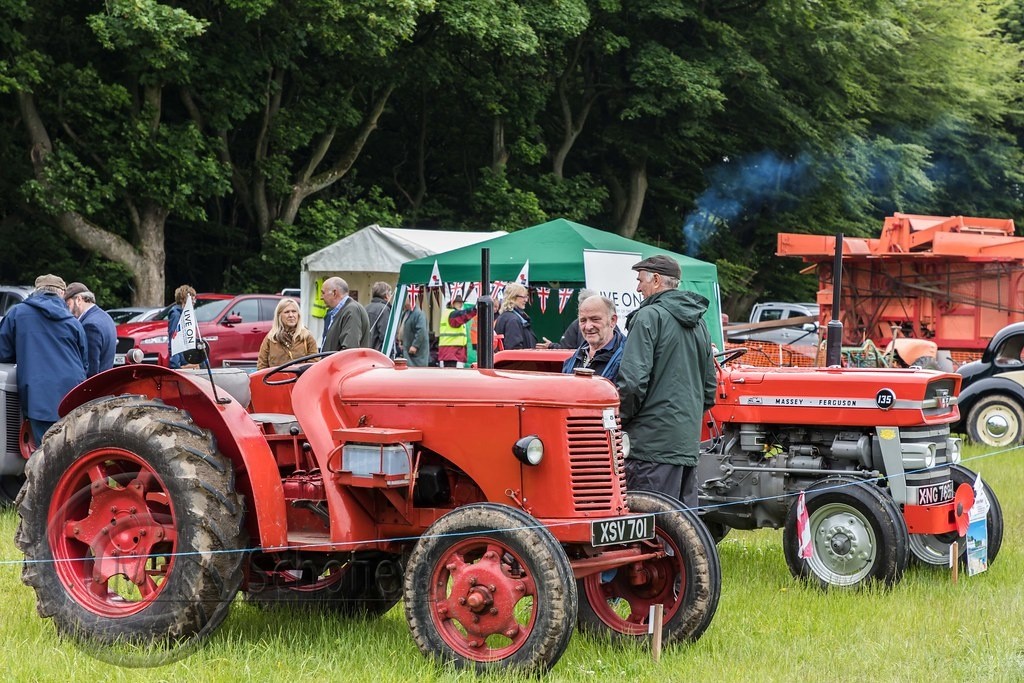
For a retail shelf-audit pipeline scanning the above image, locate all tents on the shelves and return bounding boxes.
[299,217,726,370]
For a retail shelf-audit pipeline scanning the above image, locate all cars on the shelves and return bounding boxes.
[113,292,301,370]
[0,285,300,477]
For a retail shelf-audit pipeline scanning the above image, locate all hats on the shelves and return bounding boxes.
[64,281,90,300]
[34,273,66,292]
[630,255,681,277]
[455,295,467,302]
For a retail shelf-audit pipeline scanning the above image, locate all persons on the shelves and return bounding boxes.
[257,298,321,370]
[0,274,116,446]
[365,255,718,517]
[168,285,200,369]
[320,277,370,359]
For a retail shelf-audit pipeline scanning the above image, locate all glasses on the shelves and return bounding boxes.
[516,294,529,298]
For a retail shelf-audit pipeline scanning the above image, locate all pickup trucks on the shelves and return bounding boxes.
[725,301,821,348]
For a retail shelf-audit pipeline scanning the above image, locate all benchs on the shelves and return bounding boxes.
[998,358,1021,365]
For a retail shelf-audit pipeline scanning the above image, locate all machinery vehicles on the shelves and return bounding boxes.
[12,247,724,683]
[491,228,1008,598]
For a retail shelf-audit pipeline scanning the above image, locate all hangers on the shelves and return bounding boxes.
[315,272,328,284]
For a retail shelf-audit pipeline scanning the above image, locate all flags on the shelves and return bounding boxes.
[171,295,197,356]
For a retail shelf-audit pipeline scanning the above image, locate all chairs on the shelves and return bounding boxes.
[175,367,307,435]
